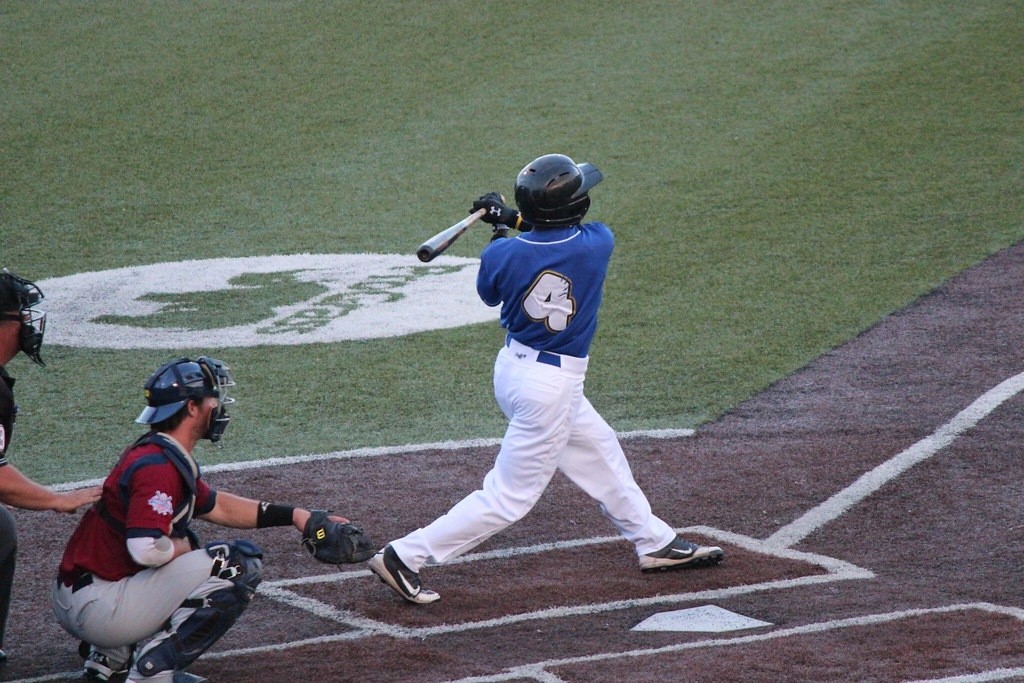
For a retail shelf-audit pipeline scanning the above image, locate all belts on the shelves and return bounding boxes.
[55,574,93,594]
[506,334,561,368]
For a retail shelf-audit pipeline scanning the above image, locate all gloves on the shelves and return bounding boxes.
[469,191,521,232]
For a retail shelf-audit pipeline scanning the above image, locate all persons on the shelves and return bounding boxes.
[52,355,378,683]
[0,271,105,663]
[369,154,724,606]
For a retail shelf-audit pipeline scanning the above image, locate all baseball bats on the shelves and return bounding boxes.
[416,194,505,263]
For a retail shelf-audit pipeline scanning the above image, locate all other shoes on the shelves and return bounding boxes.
[82,651,132,683]
[125,668,208,683]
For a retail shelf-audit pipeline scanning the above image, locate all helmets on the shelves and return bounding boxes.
[135,356,213,424]
[513,153,603,227]
[0,273,30,313]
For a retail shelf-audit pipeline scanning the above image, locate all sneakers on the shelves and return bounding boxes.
[639,535,724,572]
[368,543,441,604]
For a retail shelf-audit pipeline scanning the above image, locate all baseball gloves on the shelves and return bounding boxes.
[300,507,377,566]
